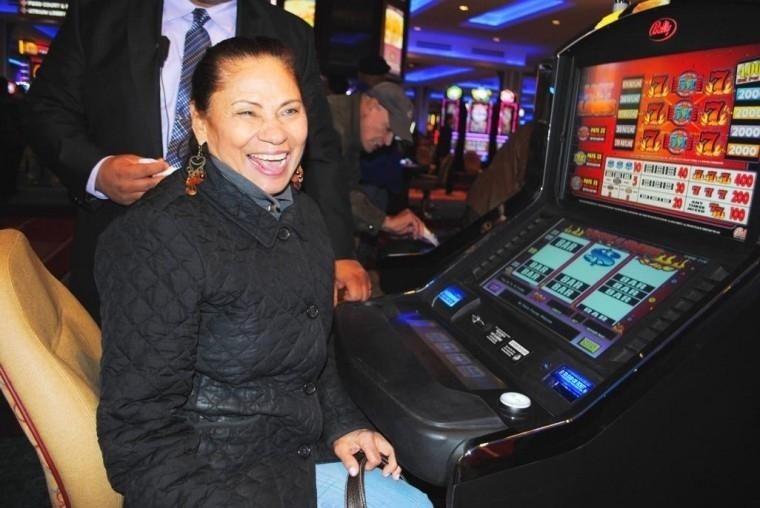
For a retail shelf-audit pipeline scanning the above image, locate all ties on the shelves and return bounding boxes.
[167,8,212,167]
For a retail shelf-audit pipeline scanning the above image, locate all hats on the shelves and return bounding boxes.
[365,82,416,146]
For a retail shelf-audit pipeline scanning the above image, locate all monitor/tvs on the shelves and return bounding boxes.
[475,215,721,365]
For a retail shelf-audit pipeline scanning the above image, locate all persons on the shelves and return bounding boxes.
[347,54,403,212]
[458,114,537,236]
[0,74,22,197]
[435,112,453,172]
[23,0,372,334]
[13,84,25,99]
[325,81,427,299]
[92,36,434,508]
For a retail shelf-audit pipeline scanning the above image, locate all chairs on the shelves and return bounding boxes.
[0,226,124,508]
